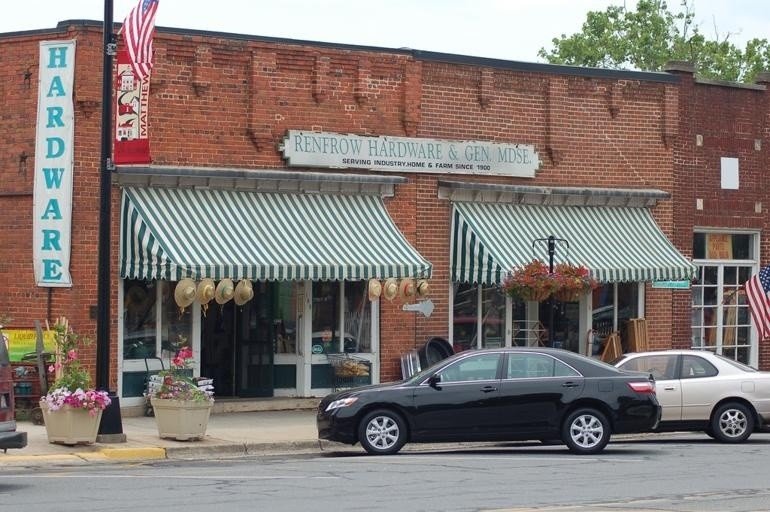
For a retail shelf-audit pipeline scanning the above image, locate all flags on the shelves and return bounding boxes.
[121,0,160,82]
[744,265,770,341]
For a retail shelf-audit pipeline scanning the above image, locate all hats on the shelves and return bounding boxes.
[368,278,382,301]
[198,277,215,305]
[174,278,197,308]
[383,278,398,300]
[215,279,234,304]
[399,279,413,301]
[234,280,254,305]
[416,279,428,298]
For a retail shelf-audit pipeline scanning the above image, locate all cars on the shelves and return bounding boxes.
[606,347,770,443]
[0,330,26,450]
[317,346,661,455]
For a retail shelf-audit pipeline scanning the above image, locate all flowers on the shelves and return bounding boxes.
[39,324,112,415]
[501,258,555,296]
[555,263,597,295]
[150,345,214,402]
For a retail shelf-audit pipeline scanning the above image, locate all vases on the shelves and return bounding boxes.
[152,398,213,440]
[41,403,102,445]
